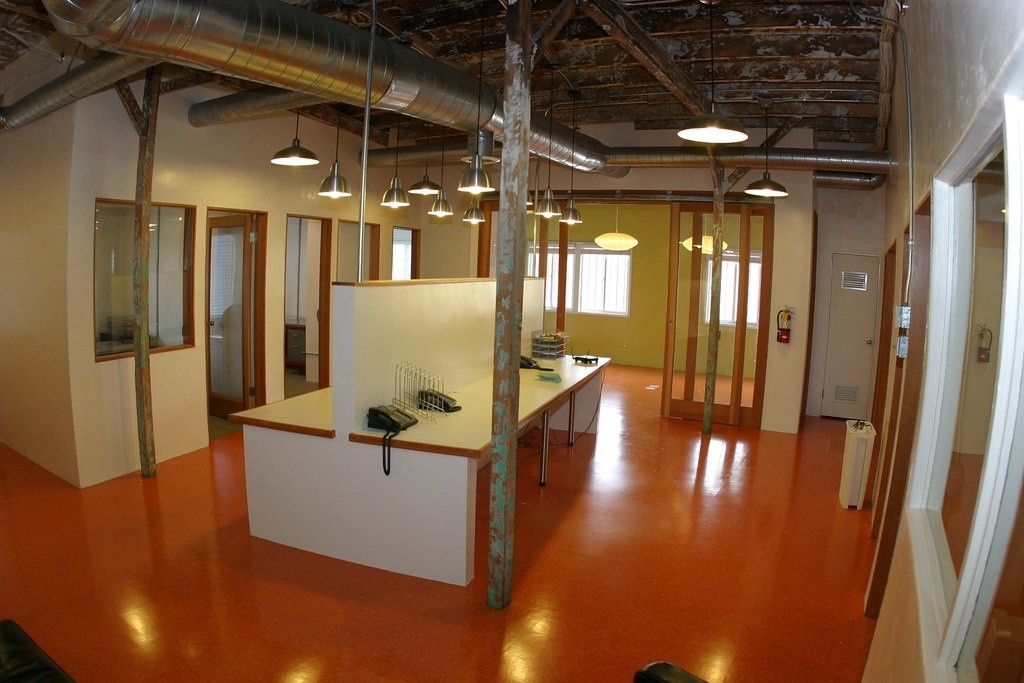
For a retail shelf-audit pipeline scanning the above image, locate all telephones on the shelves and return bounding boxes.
[520,354,537,368]
[366,403,419,432]
[417,387,457,412]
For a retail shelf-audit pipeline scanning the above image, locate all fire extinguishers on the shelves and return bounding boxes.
[978,324,992,362]
[777,305,795,343]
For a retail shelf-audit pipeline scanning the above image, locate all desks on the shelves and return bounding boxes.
[96,336,167,355]
[227,277,610,586]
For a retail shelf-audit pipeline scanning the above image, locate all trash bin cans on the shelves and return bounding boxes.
[839,417,877,511]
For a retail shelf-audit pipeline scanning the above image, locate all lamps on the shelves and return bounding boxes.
[595,203,639,252]
[682,214,728,255]
[408,122,453,217]
[318,106,352,198]
[457,0,496,224]
[745,98,788,197]
[380,114,410,209]
[527,63,583,225]
[677,0,748,144]
[271,107,320,167]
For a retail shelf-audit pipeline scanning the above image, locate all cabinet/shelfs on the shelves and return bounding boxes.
[284,325,306,374]
[531,328,572,359]
